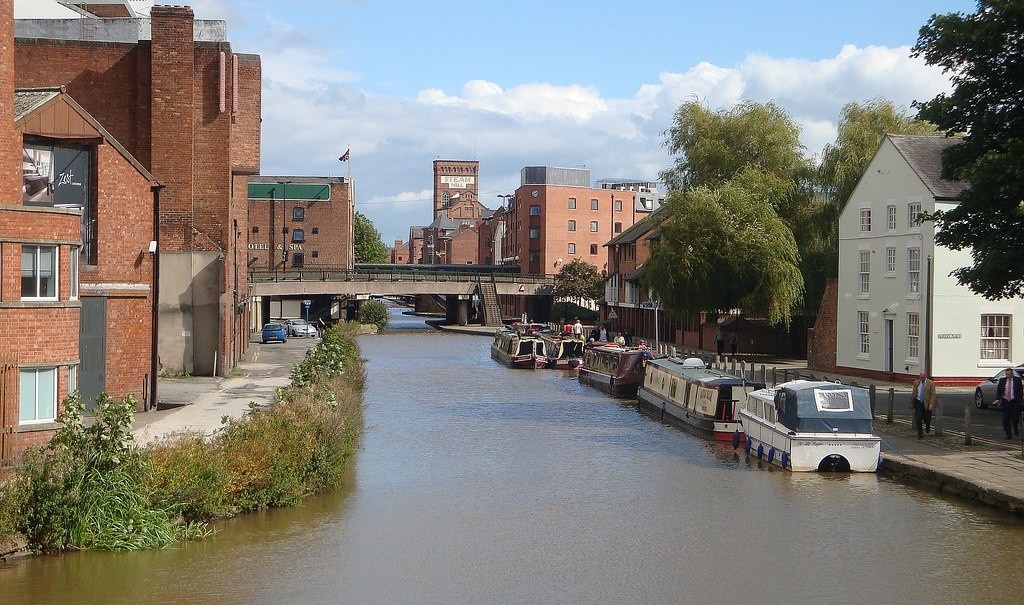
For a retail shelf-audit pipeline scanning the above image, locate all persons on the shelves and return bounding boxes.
[910,372,936,438]
[714,323,738,355]
[589,325,608,342]
[573,320,583,339]
[992,368,1022,440]
[566,322,572,334]
[614,328,648,349]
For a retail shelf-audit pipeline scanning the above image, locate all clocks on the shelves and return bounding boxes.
[531,189,539,198]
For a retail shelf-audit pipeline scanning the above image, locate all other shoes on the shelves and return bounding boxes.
[1015,429,1018,436]
[1004,436,1011,439]
[917,434,922,438]
[926,424,930,433]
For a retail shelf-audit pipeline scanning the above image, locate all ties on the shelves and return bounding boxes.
[920,382,925,403]
[1007,379,1011,402]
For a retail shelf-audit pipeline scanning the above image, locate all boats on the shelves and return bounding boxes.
[576,335,671,395]
[736,378,882,473]
[635,354,767,442]
[490,310,586,371]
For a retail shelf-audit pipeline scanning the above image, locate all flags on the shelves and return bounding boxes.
[339,149,349,161]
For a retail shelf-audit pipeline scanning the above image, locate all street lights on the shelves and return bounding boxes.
[277,180,293,280]
[496,194,512,274]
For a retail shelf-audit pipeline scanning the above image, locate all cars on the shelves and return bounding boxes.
[974,364,1024,411]
[283,319,316,338]
[260,323,288,344]
